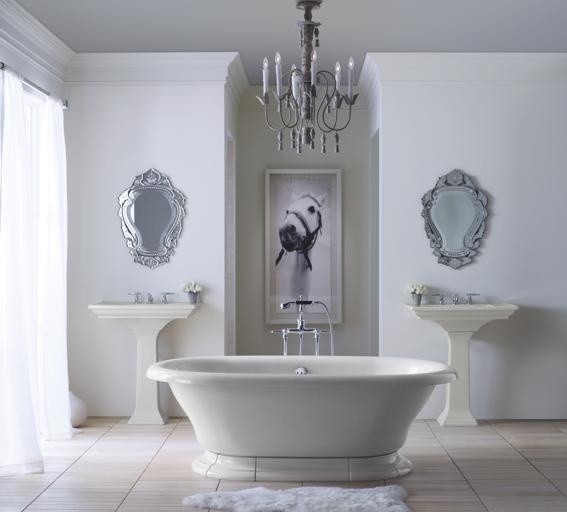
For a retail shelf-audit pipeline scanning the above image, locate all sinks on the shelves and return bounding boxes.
[86,300,206,319]
[404,302,521,321]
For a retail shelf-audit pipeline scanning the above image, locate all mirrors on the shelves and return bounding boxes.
[116,166,187,270]
[420,169,490,270]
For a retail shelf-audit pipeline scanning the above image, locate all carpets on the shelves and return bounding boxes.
[182,484,412,511]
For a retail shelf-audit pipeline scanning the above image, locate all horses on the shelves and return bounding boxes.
[275,187,330,297]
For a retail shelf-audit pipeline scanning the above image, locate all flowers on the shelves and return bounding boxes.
[179,281,203,293]
[402,281,427,296]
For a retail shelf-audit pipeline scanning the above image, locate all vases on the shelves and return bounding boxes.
[410,294,421,306]
[186,291,199,304]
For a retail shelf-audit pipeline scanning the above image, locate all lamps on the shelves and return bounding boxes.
[254,1,360,156]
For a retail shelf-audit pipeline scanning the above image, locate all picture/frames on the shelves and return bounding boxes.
[263,168,344,326]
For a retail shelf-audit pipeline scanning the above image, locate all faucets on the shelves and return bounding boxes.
[146,291,154,303]
[296,294,306,329]
[452,292,459,304]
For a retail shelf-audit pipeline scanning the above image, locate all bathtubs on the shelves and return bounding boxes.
[144,354,460,459]
